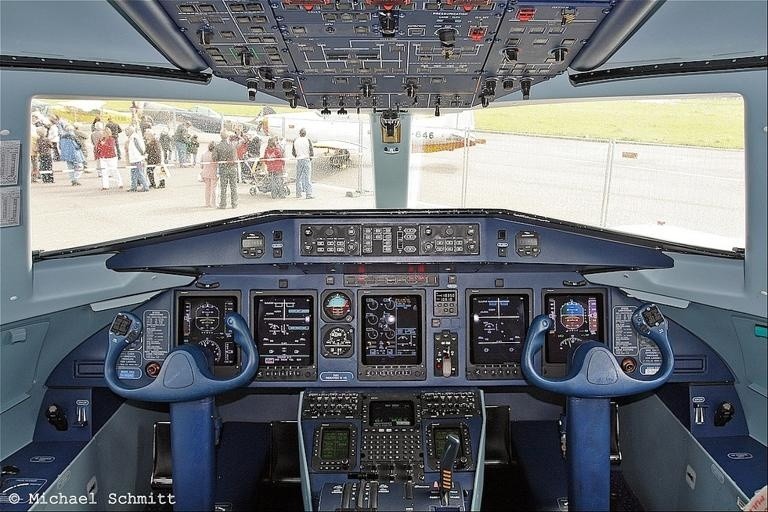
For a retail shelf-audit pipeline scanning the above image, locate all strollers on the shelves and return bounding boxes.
[246,163,291,199]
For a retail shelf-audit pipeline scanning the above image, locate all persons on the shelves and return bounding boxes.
[30,100,201,193]
[198,123,316,211]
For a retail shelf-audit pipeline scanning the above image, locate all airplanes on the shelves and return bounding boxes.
[252,107,484,170]
[131,101,276,135]
